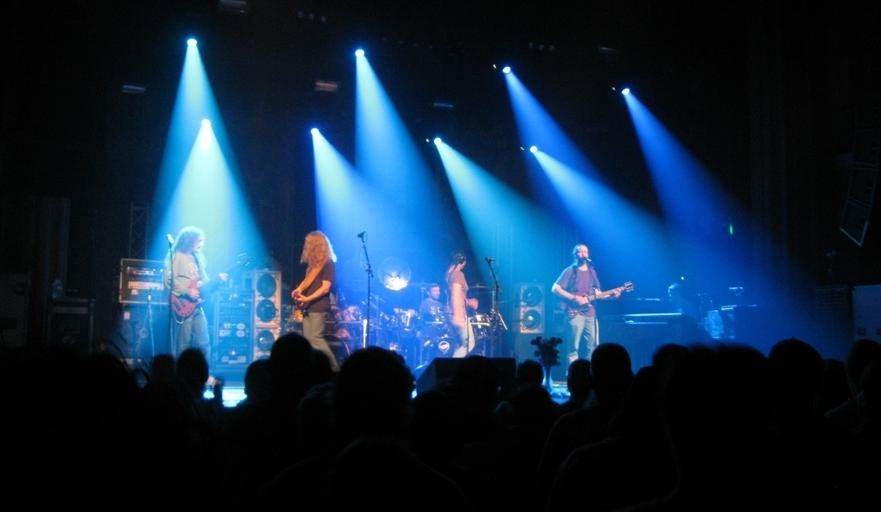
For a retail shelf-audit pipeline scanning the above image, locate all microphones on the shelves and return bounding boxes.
[485,257,495,262]
[357,231,366,237]
[166,234,174,247]
[582,257,594,265]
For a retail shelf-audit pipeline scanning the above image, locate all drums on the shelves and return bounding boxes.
[468,314,492,340]
[389,308,418,340]
[324,334,354,368]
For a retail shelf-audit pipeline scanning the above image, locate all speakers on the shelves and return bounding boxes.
[415,357,518,397]
[253,269,282,329]
[253,329,281,361]
[517,280,545,336]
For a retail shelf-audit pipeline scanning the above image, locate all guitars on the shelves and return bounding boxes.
[566,282,633,318]
[170,254,250,321]
[292,292,304,325]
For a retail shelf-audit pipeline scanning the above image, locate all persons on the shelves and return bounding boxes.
[417,282,443,324]
[292,231,340,373]
[445,252,478,358]
[0,328,881,512]
[668,283,681,298]
[551,244,625,393]
[165,227,222,388]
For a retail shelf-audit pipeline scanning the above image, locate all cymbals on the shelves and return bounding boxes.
[379,257,411,290]
